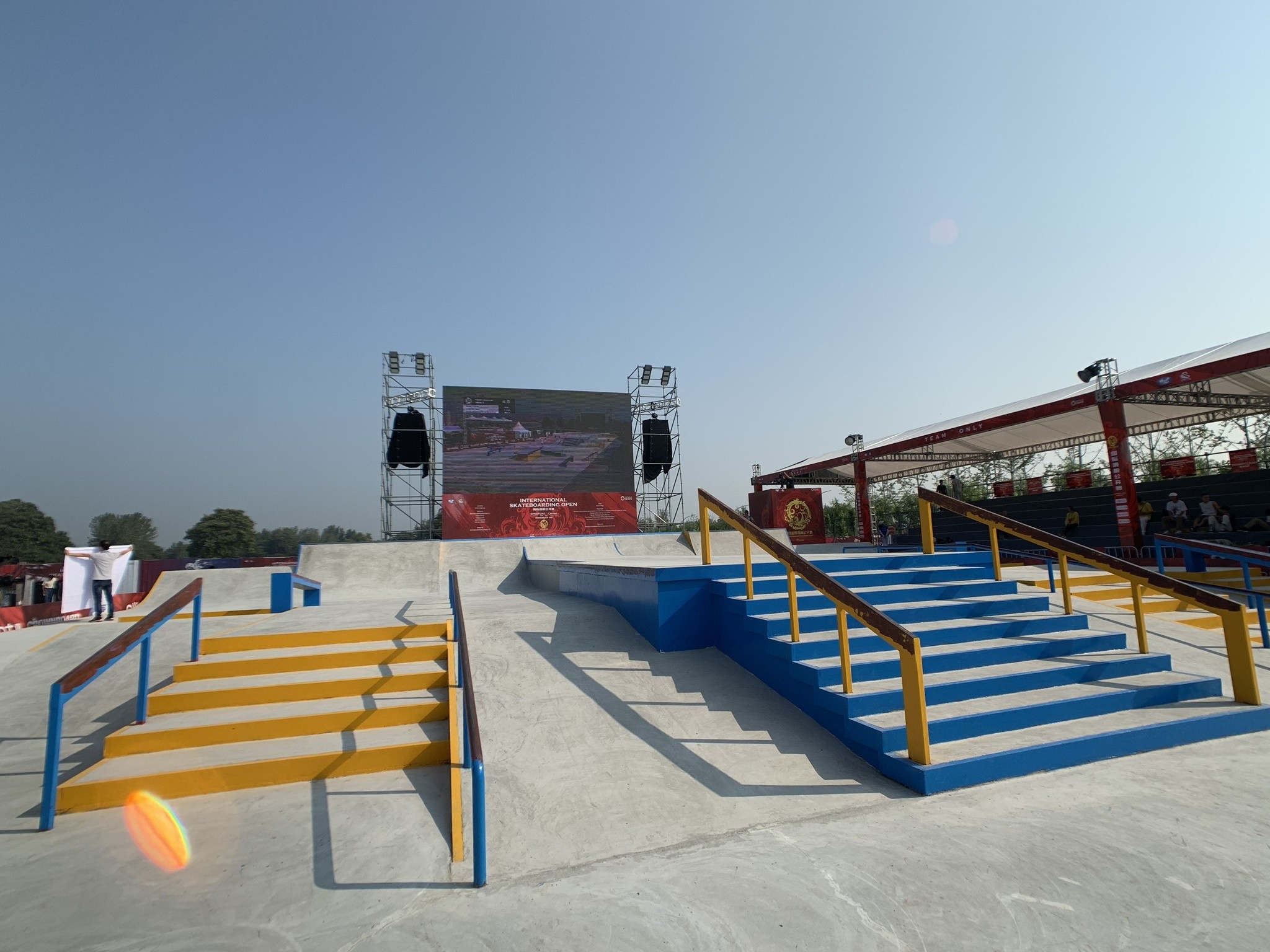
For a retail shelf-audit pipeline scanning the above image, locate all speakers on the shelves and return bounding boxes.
[642,419,669,464]
[394,413,423,461]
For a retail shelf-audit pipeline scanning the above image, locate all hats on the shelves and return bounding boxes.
[949,475,956,478]
[1169,492,1177,496]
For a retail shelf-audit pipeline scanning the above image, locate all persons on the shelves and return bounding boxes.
[42,573,63,603]
[999,510,1010,540]
[1137,495,1154,535]
[878,520,889,553]
[64,540,134,622]
[1161,493,1188,536]
[1057,505,1079,538]
[949,475,964,501]
[1190,493,1269,534]
[935,479,948,511]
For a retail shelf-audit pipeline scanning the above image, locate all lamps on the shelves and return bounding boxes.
[1077,362,1103,382]
[660,366,671,385]
[641,364,653,385]
[415,352,425,375]
[844,434,863,446]
[389,351,400,373]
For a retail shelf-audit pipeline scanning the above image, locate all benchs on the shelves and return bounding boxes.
[892,470,1270,556]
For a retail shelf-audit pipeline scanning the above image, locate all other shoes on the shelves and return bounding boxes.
[1237,527,1248,532]
[1218,526,1227,532]
[1193,525,1199,530]
[89,617,102,621]
[1180,527,1191,533]
[1175,530,1182,534]
[1060,534,1066,538]
[1209,526,1214,533]
[1163,531,1170,534]
[104,617,114,621]
